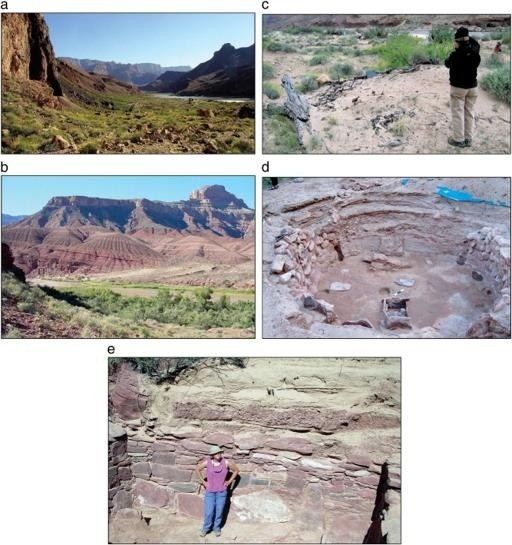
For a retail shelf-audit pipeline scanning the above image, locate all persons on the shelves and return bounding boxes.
[194,446,240,537]
[444,26,481,147]
[493,42,502,53]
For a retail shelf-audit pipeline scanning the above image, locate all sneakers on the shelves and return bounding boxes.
[448,137,472,147]
[214,529,220,537]
[200,531,206,537]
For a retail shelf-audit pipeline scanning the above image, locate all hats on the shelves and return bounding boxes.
[208,446,224,455]
[455,27,469,41]
